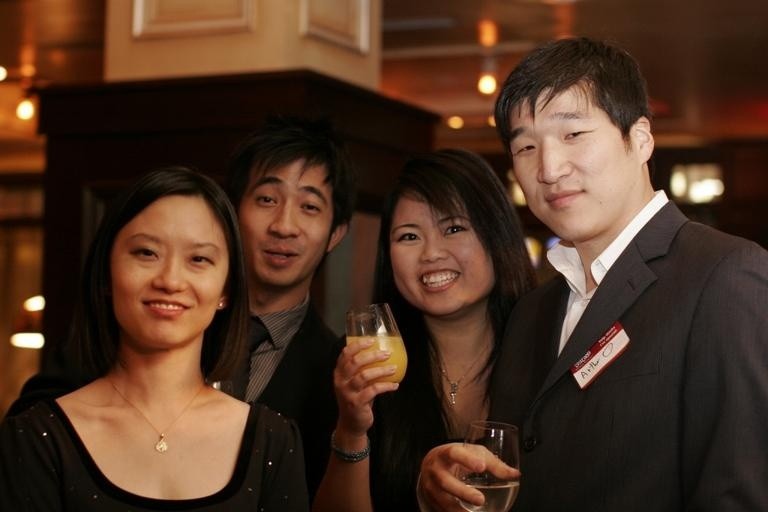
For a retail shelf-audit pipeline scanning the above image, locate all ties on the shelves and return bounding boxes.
[249,318,268,354]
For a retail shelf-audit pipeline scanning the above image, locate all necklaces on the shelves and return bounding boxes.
[434,337,490,406]
[103,374,204,456]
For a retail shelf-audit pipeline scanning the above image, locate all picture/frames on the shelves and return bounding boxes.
[299,0,370,55]
[131,0,255,40]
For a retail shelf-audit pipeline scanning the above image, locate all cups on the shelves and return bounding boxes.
[455,421,519,512]
[343,302,408,385]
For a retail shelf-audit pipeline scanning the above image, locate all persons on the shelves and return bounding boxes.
[399,32,767,511]
[0,164,315,512]
[198,125,356,511]
[309,145,540,511]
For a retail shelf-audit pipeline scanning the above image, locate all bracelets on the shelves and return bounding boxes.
[327,430,374,461]
